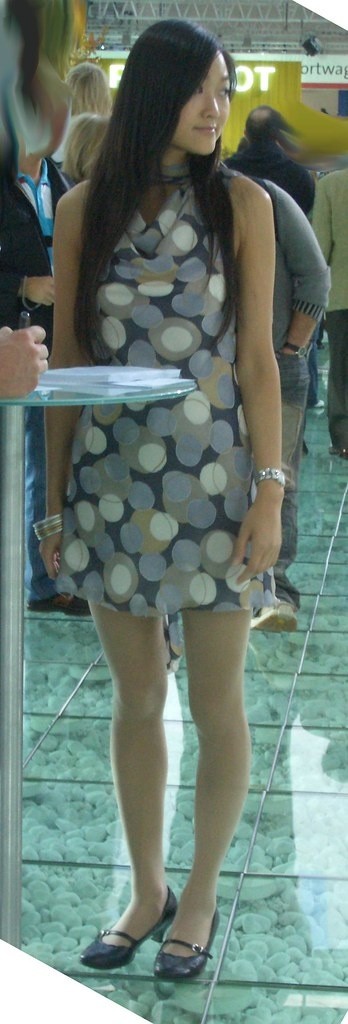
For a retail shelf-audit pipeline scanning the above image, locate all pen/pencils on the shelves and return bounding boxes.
[20,313,29,330]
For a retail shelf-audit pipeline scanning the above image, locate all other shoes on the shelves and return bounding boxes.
[249,597,297,633]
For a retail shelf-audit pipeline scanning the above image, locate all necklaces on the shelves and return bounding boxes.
[159,173,190,185]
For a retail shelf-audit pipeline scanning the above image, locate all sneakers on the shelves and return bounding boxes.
[26,592,92,616]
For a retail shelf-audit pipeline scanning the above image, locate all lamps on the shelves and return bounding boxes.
[303,37,321,57]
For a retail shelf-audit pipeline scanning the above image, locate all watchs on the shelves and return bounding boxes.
[283,341,307,358]
[254,468,286,486]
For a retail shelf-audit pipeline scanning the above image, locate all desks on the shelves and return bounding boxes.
[0,365,199,949]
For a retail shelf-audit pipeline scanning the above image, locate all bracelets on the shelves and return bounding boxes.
[32,513,63,541]
[22,275,41,310]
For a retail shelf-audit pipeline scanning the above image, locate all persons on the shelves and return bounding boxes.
[222,105,315,215]
[65,62,112,117]
[311,168,348,459]
[249,176,331,632]
[0,325,49,398]
[39,18,284,979]
[0,147,72,612]
[61,112,109,183]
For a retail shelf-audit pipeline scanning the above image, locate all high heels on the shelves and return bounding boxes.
[79,885,179,971]
[155,907,220,978]
